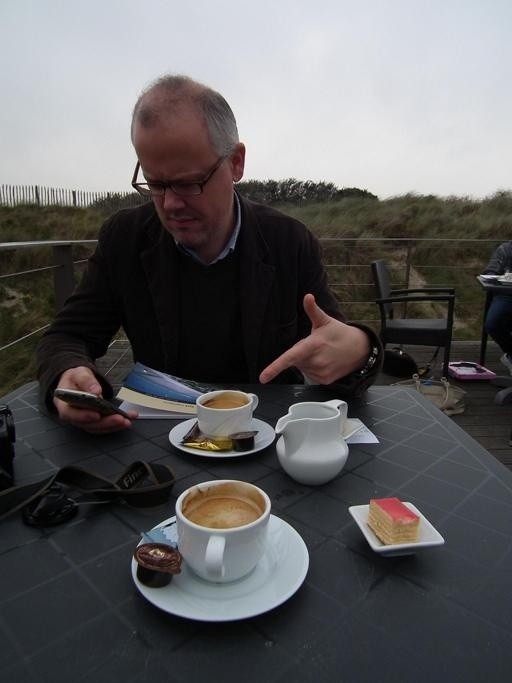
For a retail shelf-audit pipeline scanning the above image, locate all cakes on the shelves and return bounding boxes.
[366,498,420,546]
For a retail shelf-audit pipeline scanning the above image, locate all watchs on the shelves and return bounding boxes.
[360,333,381,376]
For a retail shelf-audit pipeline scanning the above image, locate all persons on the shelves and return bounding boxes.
[36,74,383,434]
[483,241,512,403]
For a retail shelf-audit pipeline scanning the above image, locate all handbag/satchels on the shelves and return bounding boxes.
[390,372,468,417]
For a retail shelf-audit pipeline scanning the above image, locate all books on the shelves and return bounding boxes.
[115,361,242,419]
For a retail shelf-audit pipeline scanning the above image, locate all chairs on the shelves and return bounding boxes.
[373,262,454,378]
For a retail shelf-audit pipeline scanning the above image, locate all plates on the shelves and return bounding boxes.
[170,416,276,458]
[349,502,446,557]
[131,514,310,621]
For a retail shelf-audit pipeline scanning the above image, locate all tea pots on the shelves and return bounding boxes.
[276,399,349,485]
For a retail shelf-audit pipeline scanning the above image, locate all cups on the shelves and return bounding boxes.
[197,390,258,441]
[175,480,271,584]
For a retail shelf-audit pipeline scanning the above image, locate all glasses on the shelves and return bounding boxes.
[132,151,231,198]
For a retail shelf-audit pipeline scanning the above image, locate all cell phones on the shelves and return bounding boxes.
[53,387,131,419]
